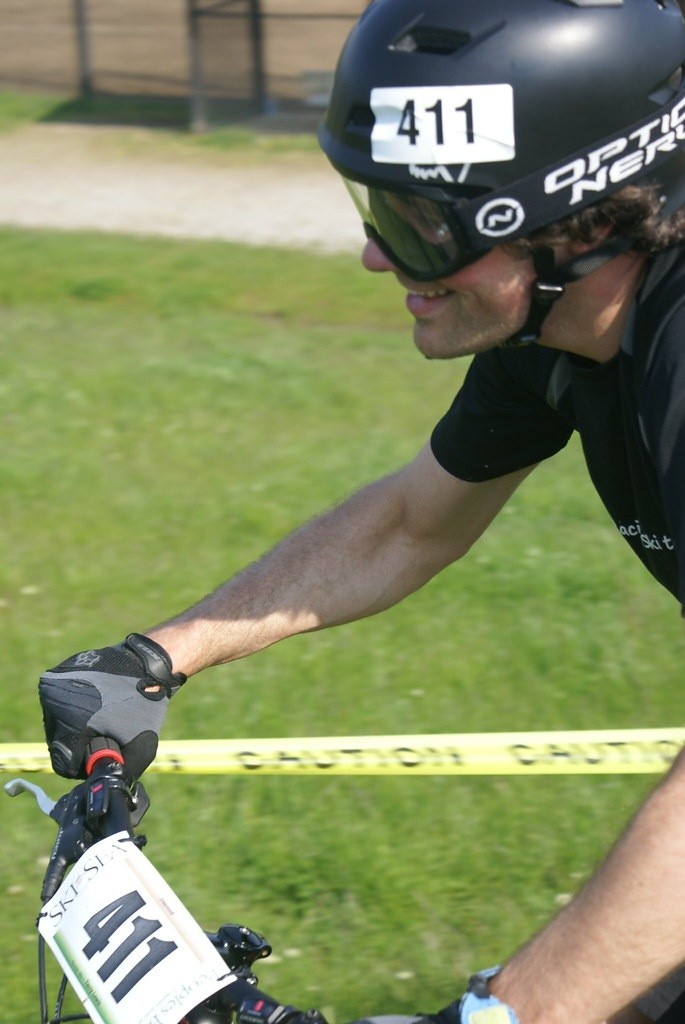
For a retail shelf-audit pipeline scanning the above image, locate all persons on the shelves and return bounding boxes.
[39,0,685,1024]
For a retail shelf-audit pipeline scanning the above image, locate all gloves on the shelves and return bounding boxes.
[348,998,460,1024]
[39,632,187,788]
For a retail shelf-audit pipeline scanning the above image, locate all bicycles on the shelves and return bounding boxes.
[2,733,358,1024]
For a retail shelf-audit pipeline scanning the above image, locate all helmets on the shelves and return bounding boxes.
[317,0,685,192]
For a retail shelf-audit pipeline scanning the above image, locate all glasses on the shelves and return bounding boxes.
[342,93,685,283]
